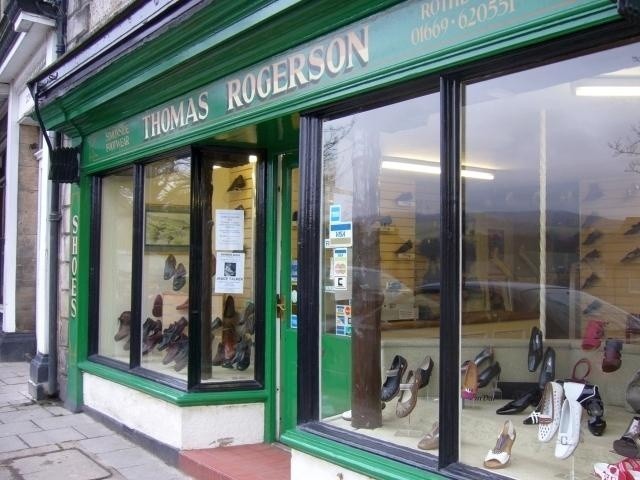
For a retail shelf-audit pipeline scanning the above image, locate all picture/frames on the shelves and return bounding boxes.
[143,204,191,253]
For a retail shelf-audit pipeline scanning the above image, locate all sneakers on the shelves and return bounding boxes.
[581,181,601,314]
[594,417,640,480]
[622,248,639,261]
[625,222,639,235]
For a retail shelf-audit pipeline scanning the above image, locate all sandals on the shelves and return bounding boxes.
[602,339,623,374]
[485,419,516,468]
[582,320,604,352]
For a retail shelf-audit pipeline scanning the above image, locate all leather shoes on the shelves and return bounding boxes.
[343,402,385,421]
[153,296,164,317]
[395,240,412,253]
[396,193,413,201]
[211,295,255,371]
[528,326,555,388]
[163,255,186,291]
[419,424,439,449]
[114,312,190,372]
[462,348,501,399]
[497,390,540,415]
[176,300,190,309]
[381,216,391,225]
[381,355,434,417]
[227,175,245,192]
[524,381,583,458]
